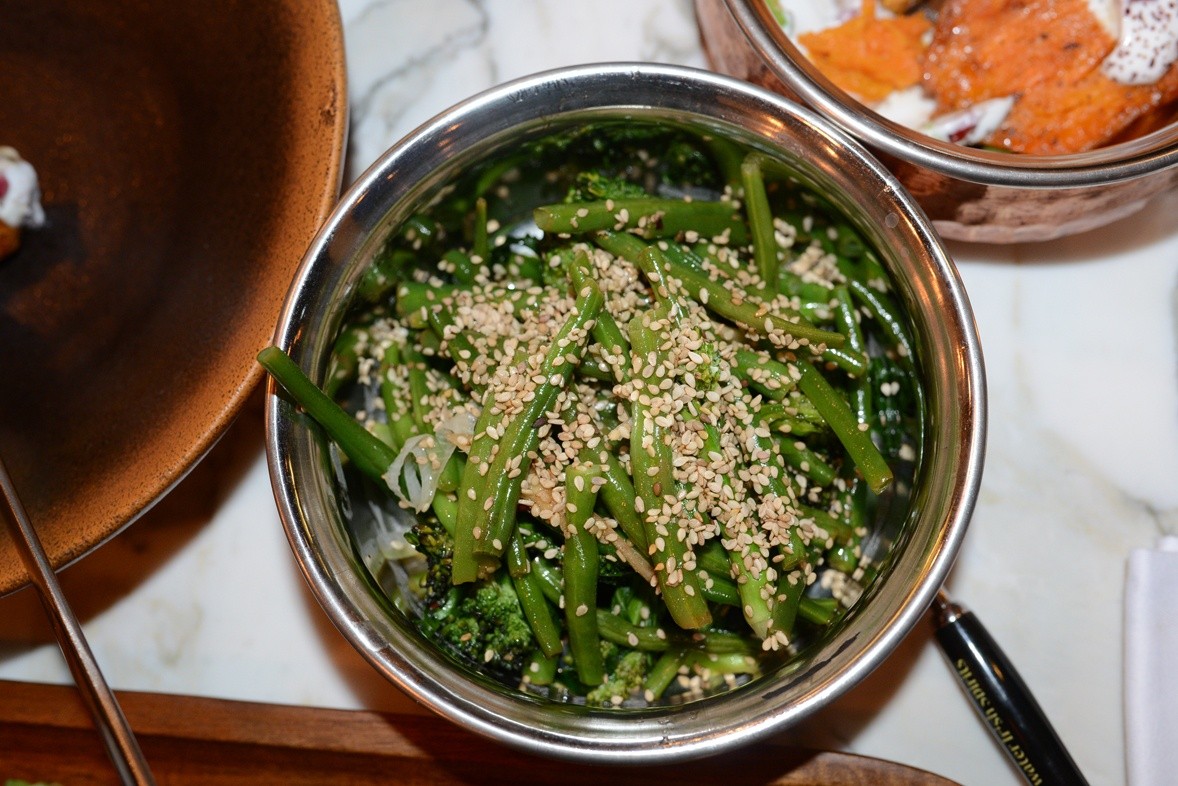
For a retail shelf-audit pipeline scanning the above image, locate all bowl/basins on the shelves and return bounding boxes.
[1,2,349,603]
[693,0,1177,243]
[261,62,988,765]
